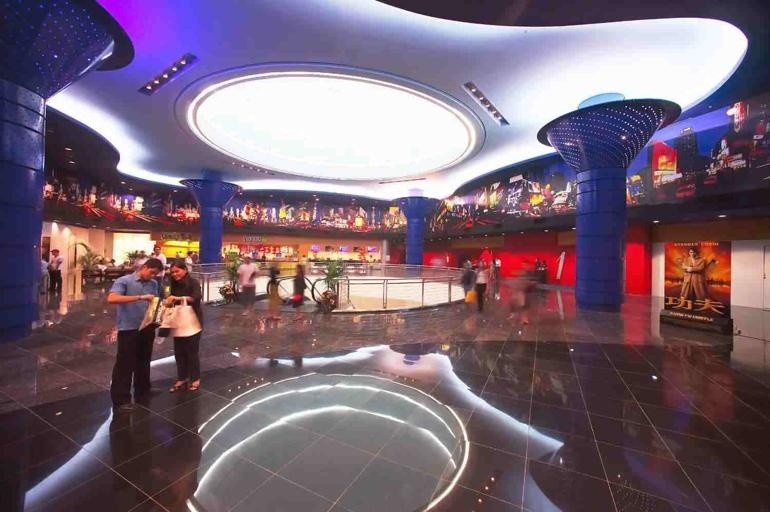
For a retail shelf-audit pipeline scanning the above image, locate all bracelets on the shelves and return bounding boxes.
[138,294,143,300]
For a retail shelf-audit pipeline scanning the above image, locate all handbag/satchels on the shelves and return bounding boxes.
[139,297,163,332]
[159,297,175,338]
[171,296,202,338]
[465,288,476,304]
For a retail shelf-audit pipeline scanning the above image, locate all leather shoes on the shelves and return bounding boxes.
[113,402,136,413]
[134,387,161,395]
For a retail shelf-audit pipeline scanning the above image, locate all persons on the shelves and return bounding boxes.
[459,255,548,325]
[150,245,167,295]
[49,249,64,293]
[293,264,306,306]
[175,249,200,272]
[267,258,282,320]
[106,257,163,411]
[40,255,51,294]
[33,293,64,329]
[158,261,205,394]
[673,247,713,313]
[133,250,148,272]
[360,254,376,276]
[236,255,260,316]
[108,394,205,511]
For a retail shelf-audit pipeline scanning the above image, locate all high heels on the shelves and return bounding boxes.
[190,379,201,391]
[169,381,187,393]
